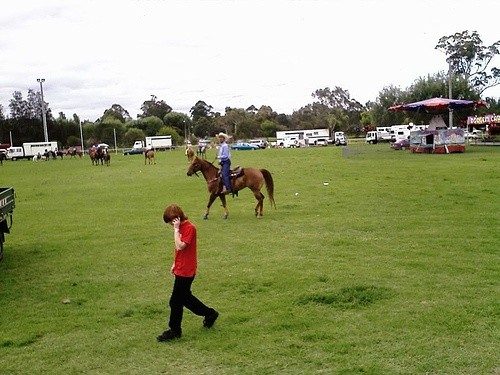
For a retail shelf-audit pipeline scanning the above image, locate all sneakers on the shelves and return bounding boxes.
[202,310,220,329]
[156,326,184,341]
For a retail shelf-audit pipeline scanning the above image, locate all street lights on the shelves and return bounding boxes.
[36,79,48,142]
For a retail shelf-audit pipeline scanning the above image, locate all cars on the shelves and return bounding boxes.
[123,147,152,156]
[390,139,409,150]
[231,142,260,152]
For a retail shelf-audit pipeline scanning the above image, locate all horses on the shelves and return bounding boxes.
[196,143,208,157]
[89,148,110,166]
[186,155,277,219]
[66,149,83,159]
[185,144,195,162]
[143,148,156,165]
[50,150,64,159]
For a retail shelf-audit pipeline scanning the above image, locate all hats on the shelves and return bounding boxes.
[216,132,233,141]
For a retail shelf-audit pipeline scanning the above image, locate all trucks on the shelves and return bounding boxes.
[390,125,429,141]
[6,140,58,161]
[365,126,392,145]
[308,131,346,147]
[131,135,172,149]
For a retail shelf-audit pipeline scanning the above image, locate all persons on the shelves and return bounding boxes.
[147,144,151,150]
[156,204,220,342]
[187,141,192,148]
[216,132,231,194]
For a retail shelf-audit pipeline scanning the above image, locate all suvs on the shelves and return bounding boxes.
[317,139,328,146]
[247,139,267,149]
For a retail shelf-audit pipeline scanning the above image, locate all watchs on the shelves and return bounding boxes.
[174,229,179,233]
